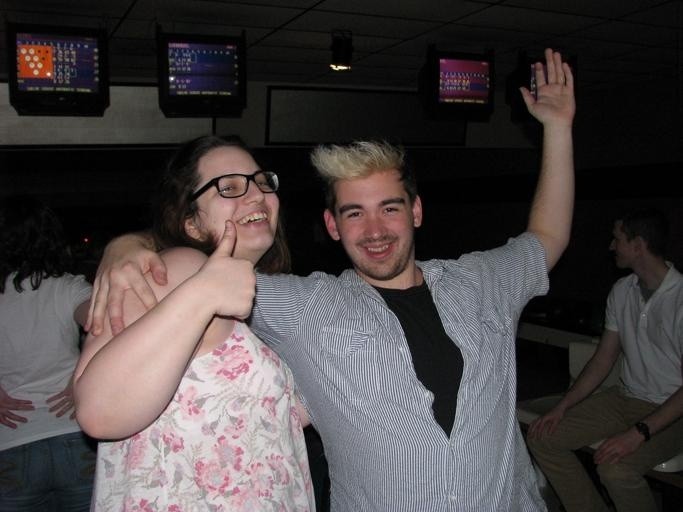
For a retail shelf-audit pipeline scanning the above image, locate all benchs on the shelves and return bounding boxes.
[517,342,681,474]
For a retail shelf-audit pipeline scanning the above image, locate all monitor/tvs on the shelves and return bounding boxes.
[422,49,496,123]
[6,21,111,117]
[156,32,247,118]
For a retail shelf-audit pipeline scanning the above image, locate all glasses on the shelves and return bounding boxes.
[191,170,280,202]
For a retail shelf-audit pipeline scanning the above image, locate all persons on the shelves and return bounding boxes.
[526,209,683,512]
[1,197,93,511]
